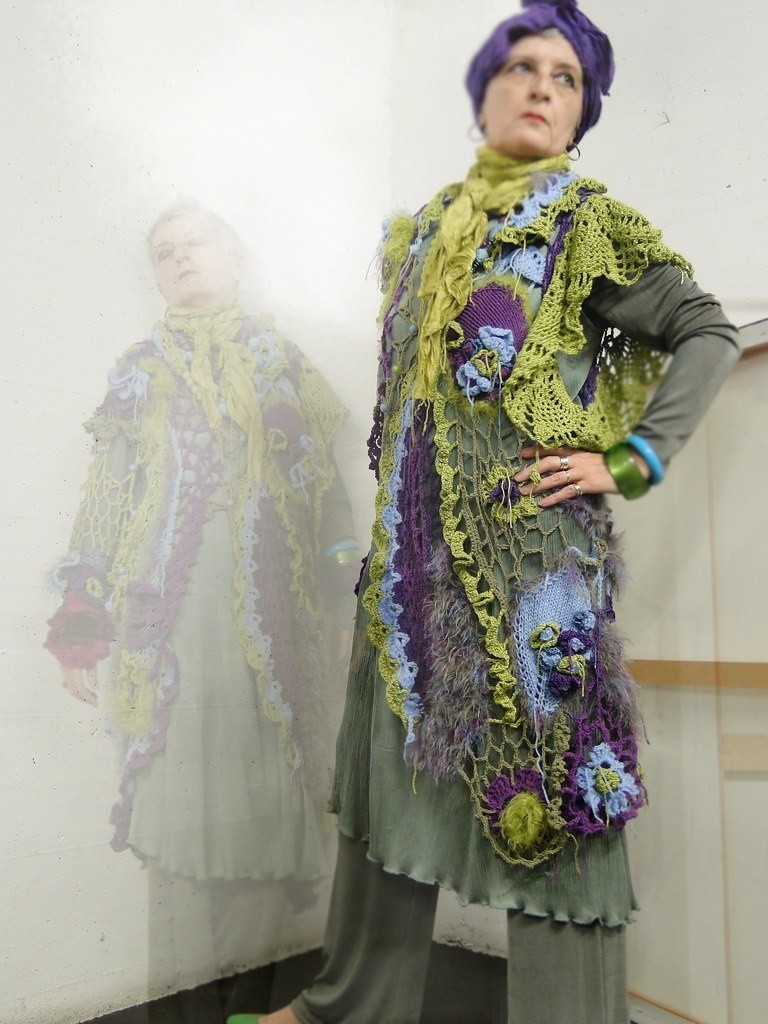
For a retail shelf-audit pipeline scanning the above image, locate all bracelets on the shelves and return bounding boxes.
[603,443,648,500]
[328,541,359,556]
[336,550,361,568]
[625,435,663,484]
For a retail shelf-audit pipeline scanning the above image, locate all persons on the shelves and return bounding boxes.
[232,1,742,1023]
[42,205,361,1023]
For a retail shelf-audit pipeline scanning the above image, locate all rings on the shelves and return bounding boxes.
[559,456,568,470]
[565,471,569,483]
[573,483,582,496]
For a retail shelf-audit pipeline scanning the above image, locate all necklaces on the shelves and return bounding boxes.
[472,240,494,272]
